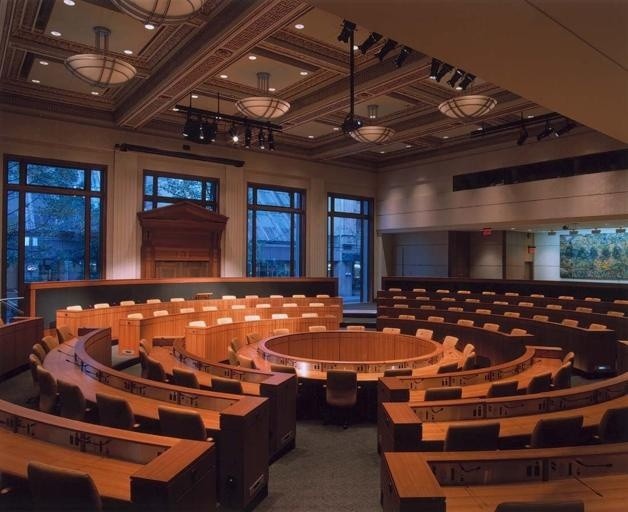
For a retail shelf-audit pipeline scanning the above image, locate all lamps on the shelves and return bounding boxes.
[517,112,577,146]
[182,113,275,152]
[349,105,396,146]
[437,82,496,119]
[337,19,477,90]
[63,26,137,88]
[340,23,363,135]
[234,72,291,121]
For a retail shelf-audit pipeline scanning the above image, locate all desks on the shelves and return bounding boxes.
[0,398,216,512]
[0,317,44,377]
